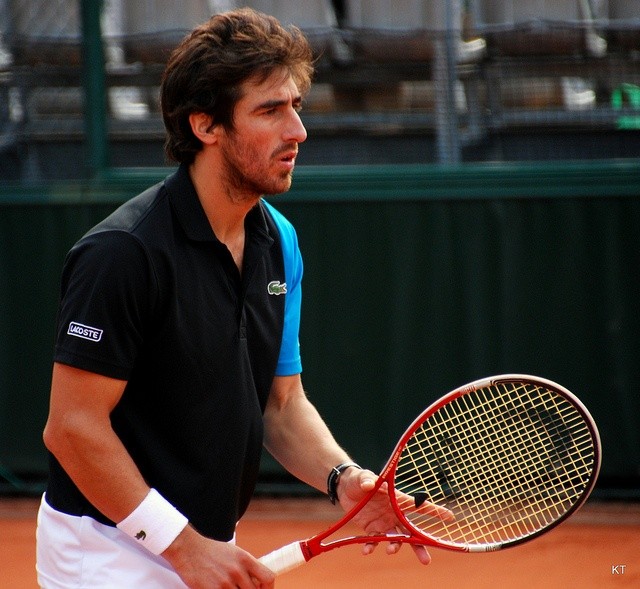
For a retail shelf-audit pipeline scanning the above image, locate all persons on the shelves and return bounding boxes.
[33,7,460,589]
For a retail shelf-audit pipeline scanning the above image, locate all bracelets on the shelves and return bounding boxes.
[324,462,362,503]
[112,487,191,556]
